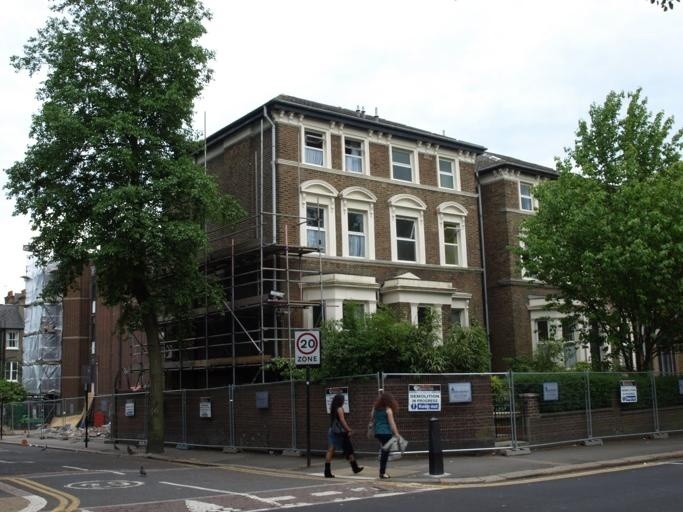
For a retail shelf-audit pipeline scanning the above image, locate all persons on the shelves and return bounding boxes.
[371,391,400,479]
[325,395,363,478]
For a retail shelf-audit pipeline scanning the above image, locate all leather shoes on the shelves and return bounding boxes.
[380,474,390,479]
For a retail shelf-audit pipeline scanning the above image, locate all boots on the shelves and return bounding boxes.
[350,460,363,473]
[325,462,334,478]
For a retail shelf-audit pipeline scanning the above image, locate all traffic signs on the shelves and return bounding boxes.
[293,329,321,366]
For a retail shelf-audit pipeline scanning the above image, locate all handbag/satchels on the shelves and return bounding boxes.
[367,421,376,440]
[332,419,346,433]
